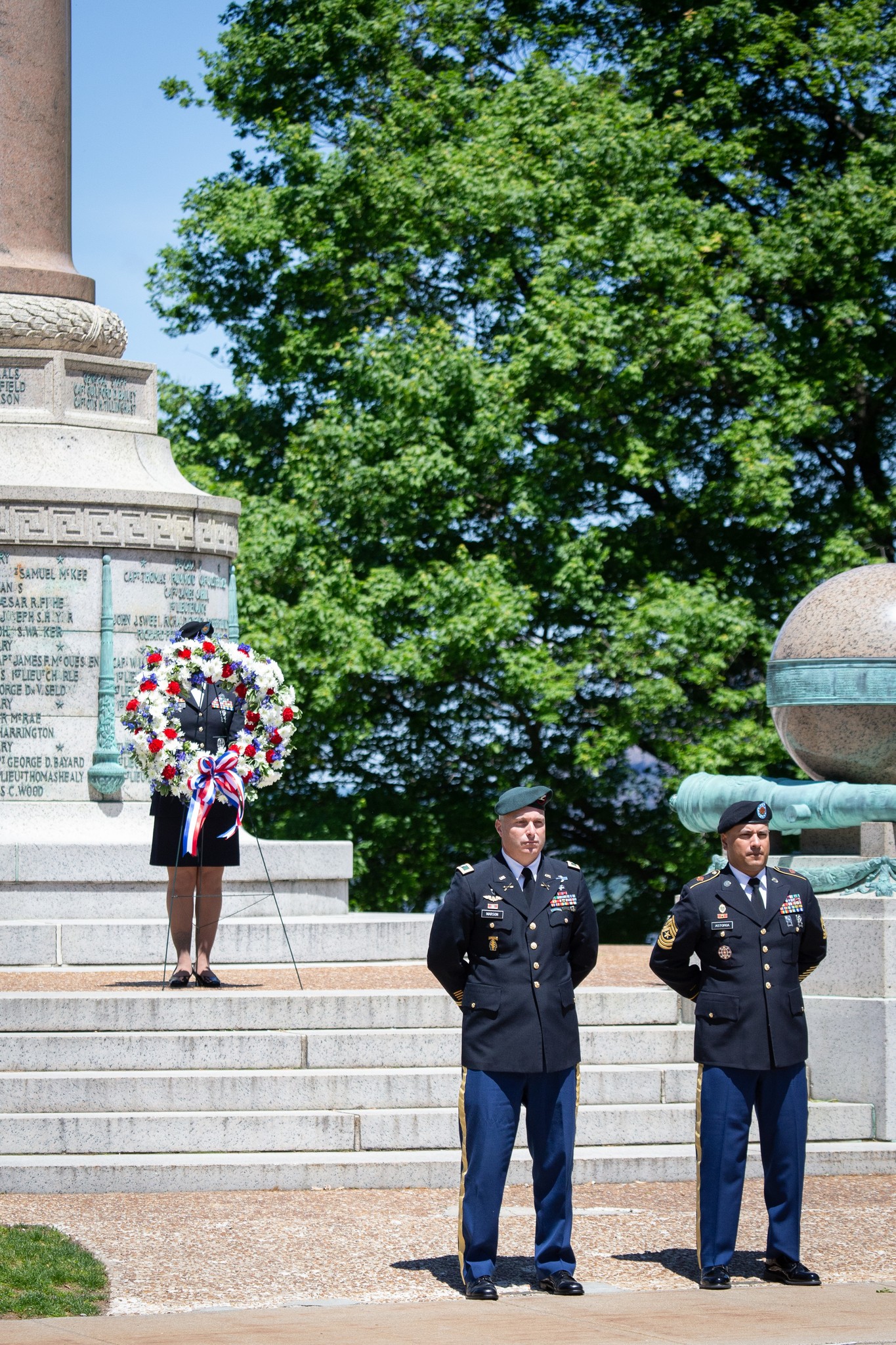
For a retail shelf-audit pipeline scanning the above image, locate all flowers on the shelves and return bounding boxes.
[120,631,302,809]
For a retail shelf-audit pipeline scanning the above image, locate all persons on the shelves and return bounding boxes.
[647,802,827,1292]
[428,787,597,1302]
[148,621,253,987]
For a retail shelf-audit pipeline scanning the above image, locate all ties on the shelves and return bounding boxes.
[747,877,765,921]
[521,868,536,904]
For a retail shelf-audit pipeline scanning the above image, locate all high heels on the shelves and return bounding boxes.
[193,962,221,986]
[168,965,193,987]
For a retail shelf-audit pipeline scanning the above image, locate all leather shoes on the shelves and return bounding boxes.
[466,1275,499,1299]
[699,1264,731,1288]
[763,1259,822,1286]
[539,1270,585,1294]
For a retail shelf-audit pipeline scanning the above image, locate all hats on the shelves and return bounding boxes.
[175,621,214,641]
[495,786,553,815]
[717,801,772,834]
